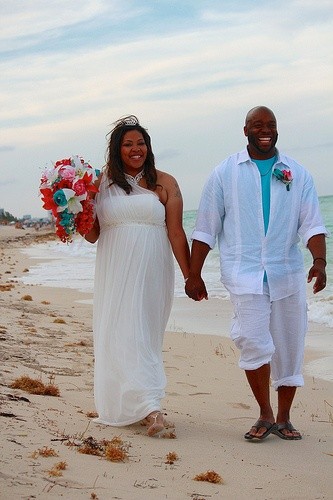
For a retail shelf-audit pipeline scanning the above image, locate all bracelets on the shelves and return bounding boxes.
[312,257,328,267]
[184,277,189,282]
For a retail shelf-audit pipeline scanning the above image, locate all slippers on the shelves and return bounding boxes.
[273,423,302,440]
[245,422,278,441]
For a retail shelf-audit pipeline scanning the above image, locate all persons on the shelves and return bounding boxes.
[187,105,329,443]
[42,116,206,436]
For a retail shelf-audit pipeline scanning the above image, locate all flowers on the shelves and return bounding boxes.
[273,167,294,192]
[38,152,104,245]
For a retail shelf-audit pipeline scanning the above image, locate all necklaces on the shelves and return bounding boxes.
[260,153,276,176]
[123,169,145,186]
